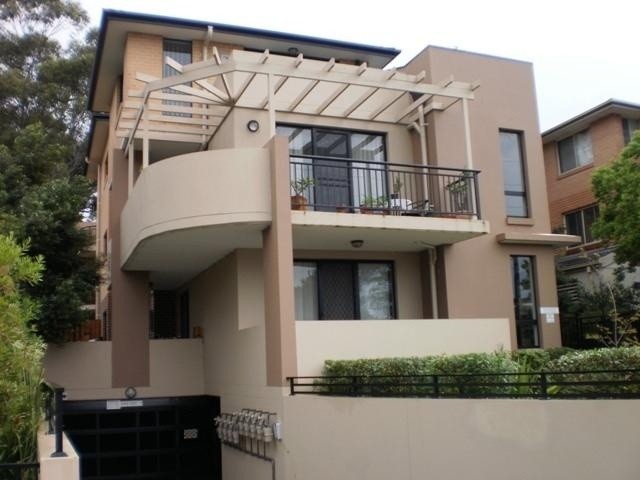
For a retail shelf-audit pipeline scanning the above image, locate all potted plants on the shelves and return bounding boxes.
[336,203,348,212]
[360,176,403,215]
[291,174,320,211]
